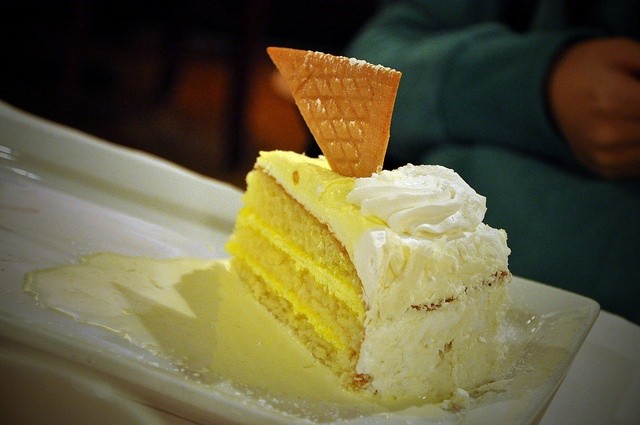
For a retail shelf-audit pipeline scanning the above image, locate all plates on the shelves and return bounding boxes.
[1,103,601,425]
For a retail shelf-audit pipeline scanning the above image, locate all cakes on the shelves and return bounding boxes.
[224,45,512,403]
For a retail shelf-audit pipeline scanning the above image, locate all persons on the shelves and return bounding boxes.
[346,0,636,289]
